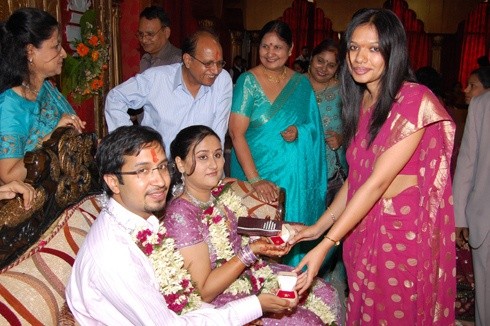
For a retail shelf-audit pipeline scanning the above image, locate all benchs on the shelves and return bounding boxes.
[0,122,286,326]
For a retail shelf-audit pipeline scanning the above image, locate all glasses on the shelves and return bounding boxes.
[136,29,162,40]
[192,56,225,68]
[120,159,170,180]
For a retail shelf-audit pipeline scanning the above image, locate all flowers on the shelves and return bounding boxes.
[59,6,111,102]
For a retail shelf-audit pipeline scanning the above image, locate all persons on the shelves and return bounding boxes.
[281,7,456,326]
[302,39,346,296]
[230,55,247,86]
[415,54,490,326]
[293,60,308,73]
[0,6,86,186]
[157,125,343,326]
[0,180,36,210]
[296,45,308,62]
[64,126,299,326]
[104,5,327,279]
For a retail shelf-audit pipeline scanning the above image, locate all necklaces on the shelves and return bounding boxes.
[103,208,202,315]
[23,81,38,95]
[187,194,279,294]
[264,65,287,86]
[311,75,331,103]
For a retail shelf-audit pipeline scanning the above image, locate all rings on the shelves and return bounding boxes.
[333,147,335,150]
[71,116,76,120]
[288,308,291,312]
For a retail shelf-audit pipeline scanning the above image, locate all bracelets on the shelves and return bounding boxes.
[324,236,340,246]
[249,177,262,184]
[236,245,259,267]
[327,207,335,222]
